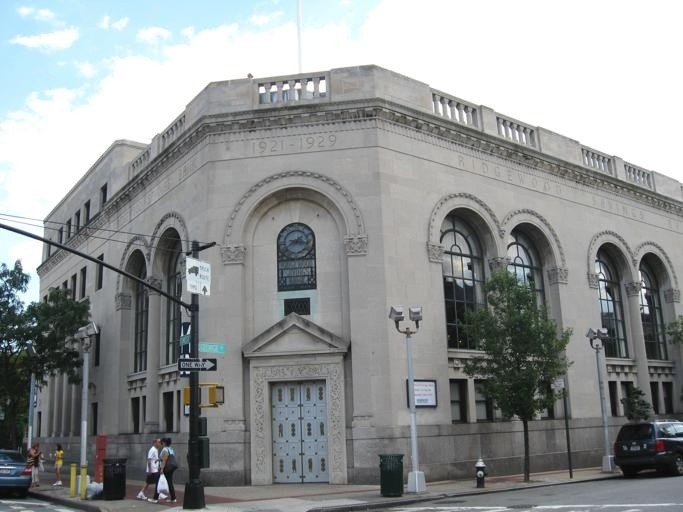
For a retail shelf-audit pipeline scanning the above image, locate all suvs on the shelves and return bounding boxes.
[614,419,683,478]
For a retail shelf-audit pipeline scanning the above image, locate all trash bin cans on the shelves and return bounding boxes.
[378,454,405,497]
[102,458,128,500]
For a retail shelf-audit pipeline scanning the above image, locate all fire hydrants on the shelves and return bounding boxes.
[474,455,488,490]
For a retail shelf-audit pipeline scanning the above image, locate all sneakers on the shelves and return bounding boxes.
[58,481,63,485]
[148,499,158,503]
[165,499,176,503]
[159,494,168,499]
[53,482,57,485]
[136,493,147,499]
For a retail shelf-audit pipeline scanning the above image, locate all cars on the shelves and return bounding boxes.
[0,449,33,497]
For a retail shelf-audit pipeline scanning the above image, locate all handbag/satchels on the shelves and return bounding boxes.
[162,449,178,475]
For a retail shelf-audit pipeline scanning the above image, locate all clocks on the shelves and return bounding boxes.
[275,221,315,261]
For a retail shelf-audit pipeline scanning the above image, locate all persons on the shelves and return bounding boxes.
[148,438,177,504]
[136,439,162,500]
[26,443,64,487]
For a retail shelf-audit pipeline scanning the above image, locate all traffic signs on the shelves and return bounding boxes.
[178,359,217,372]
[198,342,225,354]
[178,333,190,347]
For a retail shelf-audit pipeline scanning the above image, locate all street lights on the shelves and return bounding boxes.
[72,322,97,492]
[586,325,616,473]
[387,304,434,494]
